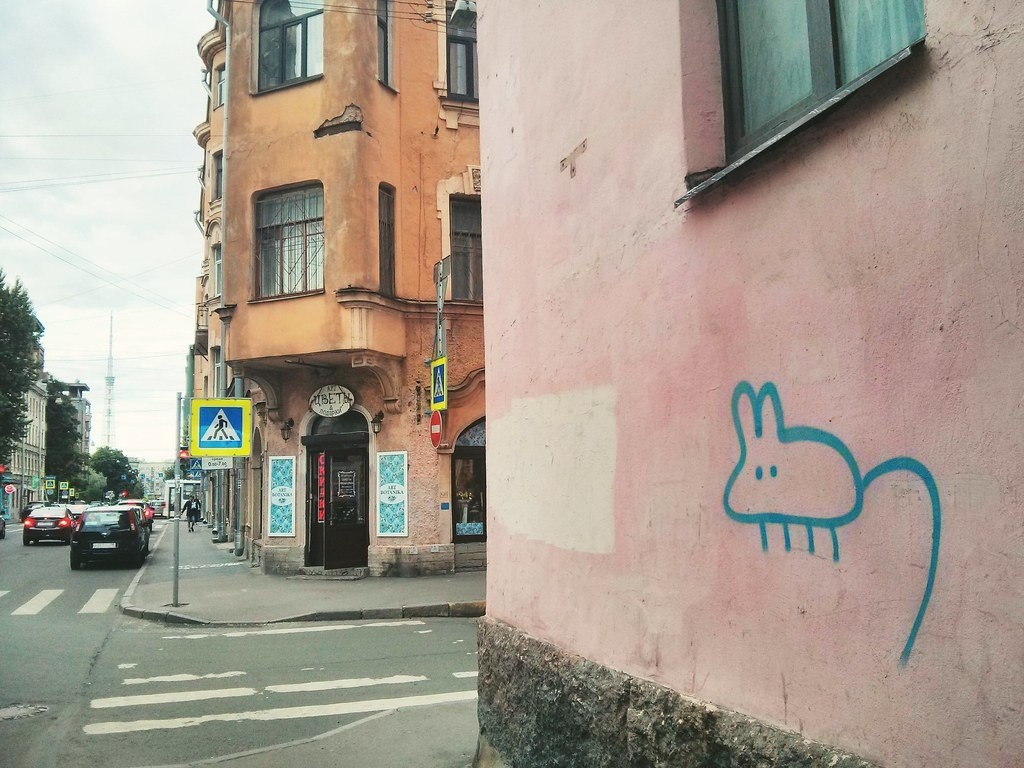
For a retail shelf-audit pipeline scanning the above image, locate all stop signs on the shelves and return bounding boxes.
[430,410,442,448]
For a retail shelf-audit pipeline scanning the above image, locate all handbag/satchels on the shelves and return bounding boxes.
[191,502,197,509]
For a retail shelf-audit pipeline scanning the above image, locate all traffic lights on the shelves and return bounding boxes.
[179,446,189,471]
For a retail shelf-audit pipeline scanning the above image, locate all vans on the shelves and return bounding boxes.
[22,500,51,522]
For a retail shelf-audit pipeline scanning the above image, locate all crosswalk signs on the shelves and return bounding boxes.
[193,472,205,479]
[433,364,445,403]
[62,483,67,488]
[199,406,242,448]
[190,459,202,472]
[47,481,53,487]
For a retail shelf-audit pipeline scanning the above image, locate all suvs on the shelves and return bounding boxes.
[116,499,154,532]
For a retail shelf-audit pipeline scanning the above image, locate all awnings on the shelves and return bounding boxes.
[0,472,22,484]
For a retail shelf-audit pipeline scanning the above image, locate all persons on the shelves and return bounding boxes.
[180,494,201,533]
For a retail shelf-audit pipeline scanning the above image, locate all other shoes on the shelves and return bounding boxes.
[189,530,190,532]
[191,527,194,532]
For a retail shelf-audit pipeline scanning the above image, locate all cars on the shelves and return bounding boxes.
[66,504,93,520]
[0,515,6,539]
[149,498,167,519]
[51,501,110,507]
[22,506,76,546]
[70,506,151,570]
